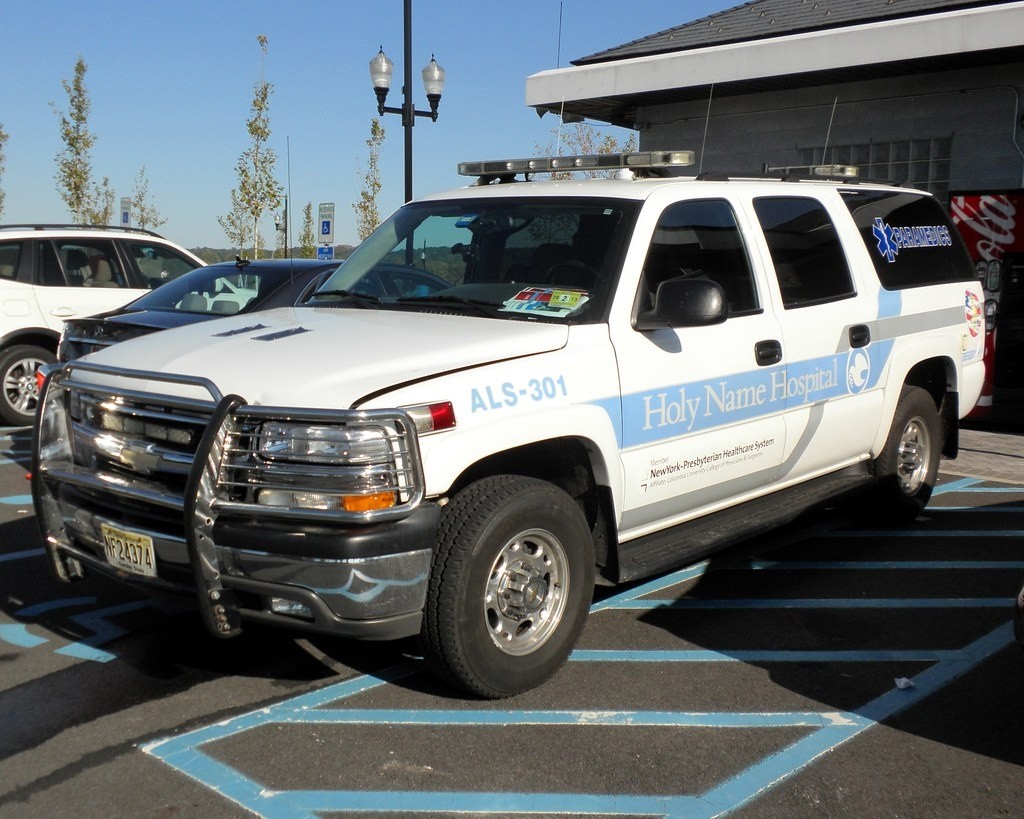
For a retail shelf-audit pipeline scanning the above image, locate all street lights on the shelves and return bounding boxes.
[420,239,427,271]
[368,1,446,294]
[273,194,289,258]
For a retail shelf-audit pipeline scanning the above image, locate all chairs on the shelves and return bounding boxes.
[58,248,88,287]
[80,255,119,287]
[504,243,597,290]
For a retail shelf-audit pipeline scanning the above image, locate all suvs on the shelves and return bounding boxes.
[0,221,258,427]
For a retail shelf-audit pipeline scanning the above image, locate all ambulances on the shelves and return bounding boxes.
[28,148,990,701]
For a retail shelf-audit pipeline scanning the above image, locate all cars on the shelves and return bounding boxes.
[36,258,455,392]
